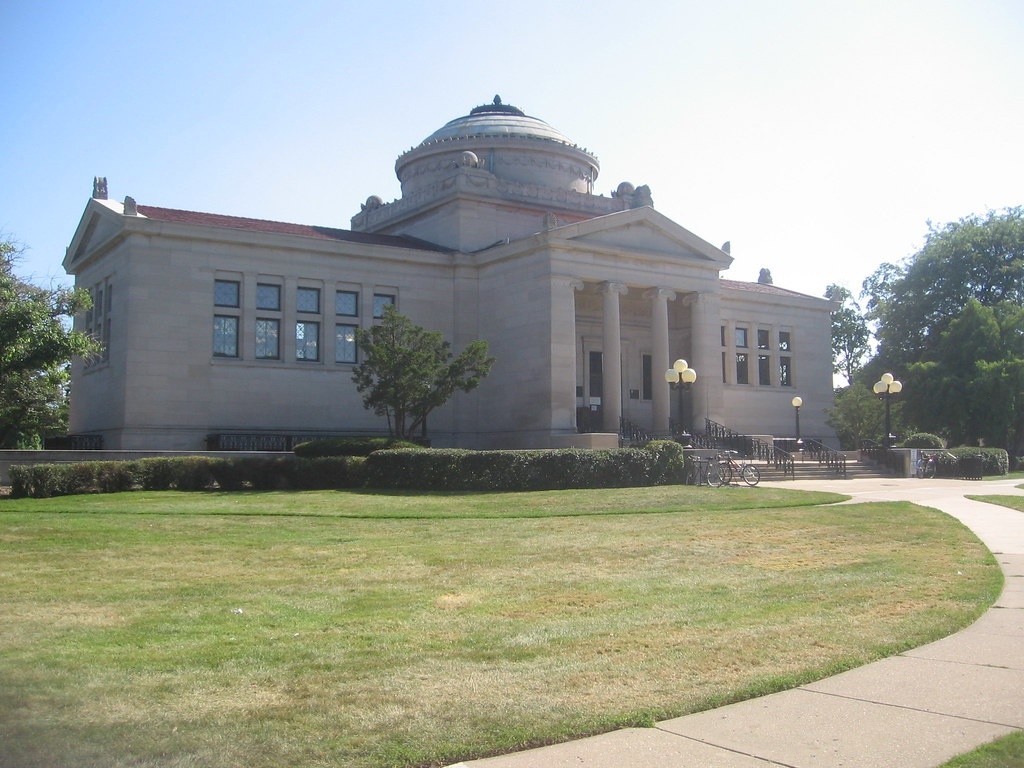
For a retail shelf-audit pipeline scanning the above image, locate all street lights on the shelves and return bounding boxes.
[873,372,903,448]
[792,396,802,452]
[664,359,697,426]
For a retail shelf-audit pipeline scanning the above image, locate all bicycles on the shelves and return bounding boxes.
[685,450,760,488]
[916,451,938,479]
